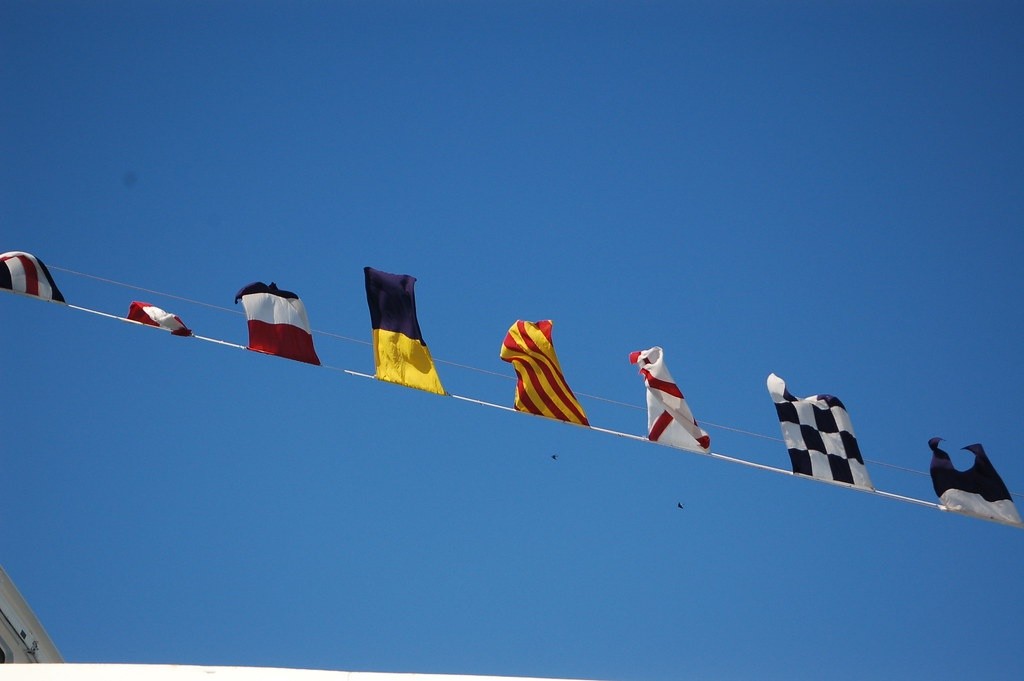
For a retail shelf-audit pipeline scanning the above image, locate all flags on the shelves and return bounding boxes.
[127,301,192,336]
[630,347,709,454]
[0,251,66,303]
[766,373,873,489]
[500,320,590,425]
[235,281,320,366]
[928,437,1021,524]
[364,267,445,395]
